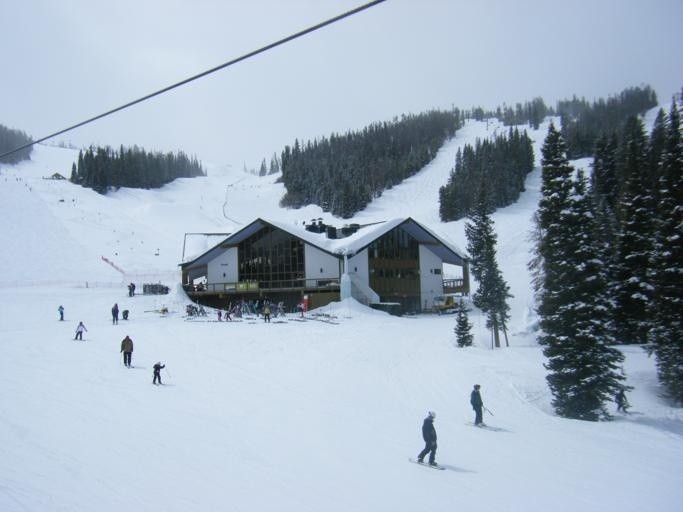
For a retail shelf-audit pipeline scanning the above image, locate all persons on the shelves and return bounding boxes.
[128,283,135,297]
[614,389,627,413]
[112,303,119,325]
[471,384,485,427]
[120,336,133,366]
[417,411,438,465]
[196,282,203,291]
[159,298,304,322]
[75,322,87,340]
[122,310,129,319]
[58,306,64,320]
[152,362,165,384]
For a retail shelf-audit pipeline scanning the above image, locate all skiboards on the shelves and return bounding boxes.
[125,362,135,370]
[468,420,503,433]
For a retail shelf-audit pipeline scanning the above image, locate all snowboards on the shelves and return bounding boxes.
[408,457,446,471]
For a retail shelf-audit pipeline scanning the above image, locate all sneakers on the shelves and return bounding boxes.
[417,457,437,466]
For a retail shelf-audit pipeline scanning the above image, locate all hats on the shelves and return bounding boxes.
[474,385,480,389]
[428,411,435,416]
